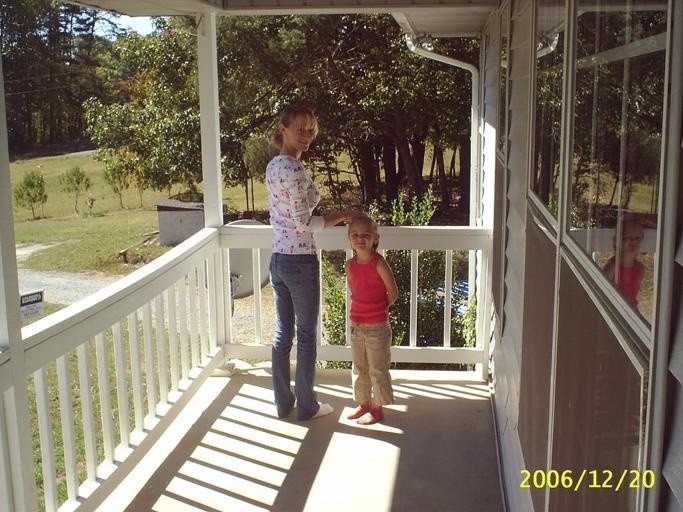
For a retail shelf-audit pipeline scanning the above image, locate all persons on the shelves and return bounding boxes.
[344,215,401,426]
[618,210,656,230]
[598,217,643,314]
[263,104,366,421]
